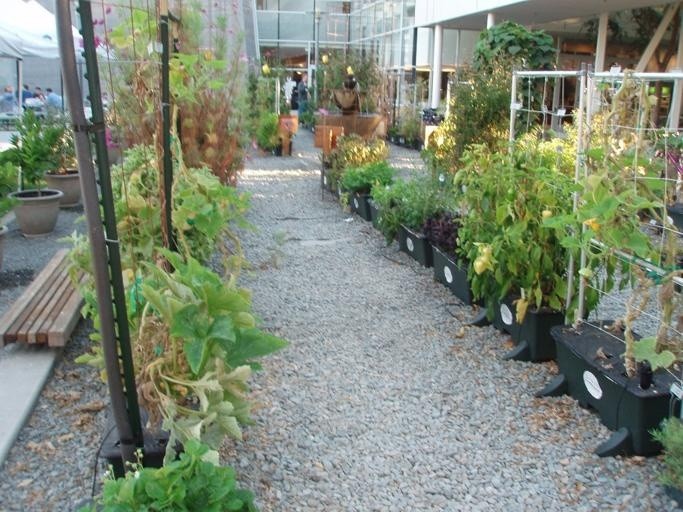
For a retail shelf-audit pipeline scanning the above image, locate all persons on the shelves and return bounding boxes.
[30,87,46,122]
[0,85,24,114]
[20,84,32,109]
[296,72,311,128]
[38,88,63,121]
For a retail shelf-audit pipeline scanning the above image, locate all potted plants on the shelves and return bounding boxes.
[0,105,80,275]
[316,45,683,458]
[268,133,282,157]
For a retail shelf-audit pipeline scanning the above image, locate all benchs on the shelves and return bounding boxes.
[0,247,87,348]
